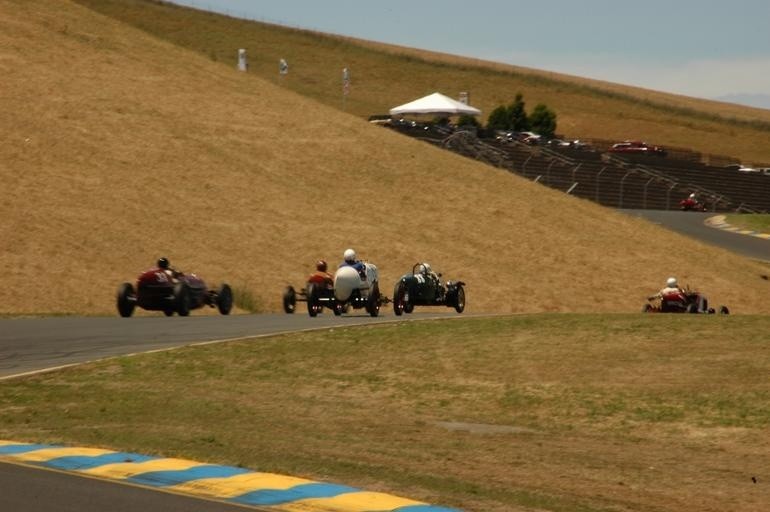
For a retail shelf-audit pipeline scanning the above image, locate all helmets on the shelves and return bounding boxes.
[667,277,676,287]
[343,249,356,262]
[158,258,167,268]
[420,263,432,274]
[316,261,327,271]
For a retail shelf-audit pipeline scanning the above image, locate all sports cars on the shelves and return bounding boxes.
[682,206,707,213]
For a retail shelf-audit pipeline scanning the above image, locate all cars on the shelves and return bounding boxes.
[278,257,383,322]
[723,162,770,176]
[640,287,711,314]
[391,262,468,317]
[112,258,238,316]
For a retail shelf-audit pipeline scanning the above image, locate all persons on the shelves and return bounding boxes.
[667,277,694,301]
[338,249,366,278]
[420,262,443,285]
[315,258,336,288]
[156,257,184,277]
[691,193,700,206]
